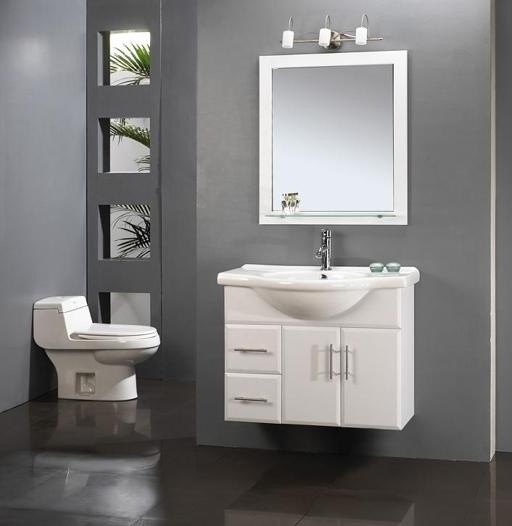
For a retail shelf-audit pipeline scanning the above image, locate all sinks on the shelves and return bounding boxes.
[217,263,419,321]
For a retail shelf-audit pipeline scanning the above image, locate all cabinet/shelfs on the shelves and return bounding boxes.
[223,285,416,433]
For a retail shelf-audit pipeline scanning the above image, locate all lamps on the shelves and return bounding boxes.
[279,15,384,50]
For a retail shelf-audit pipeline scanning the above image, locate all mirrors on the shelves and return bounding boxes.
[257,51,408,226]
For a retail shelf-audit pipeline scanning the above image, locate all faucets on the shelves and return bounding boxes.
[314,230,332,269]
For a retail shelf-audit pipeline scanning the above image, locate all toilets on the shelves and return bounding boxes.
[33,399,162,498]
[32,295,161,401]
